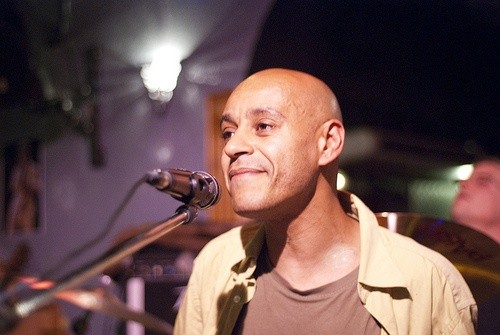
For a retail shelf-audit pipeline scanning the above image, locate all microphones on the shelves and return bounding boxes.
[145,168,222,209]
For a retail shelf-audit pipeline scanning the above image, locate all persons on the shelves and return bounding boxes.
[172,68,479,335]
[451,158,500,243]
